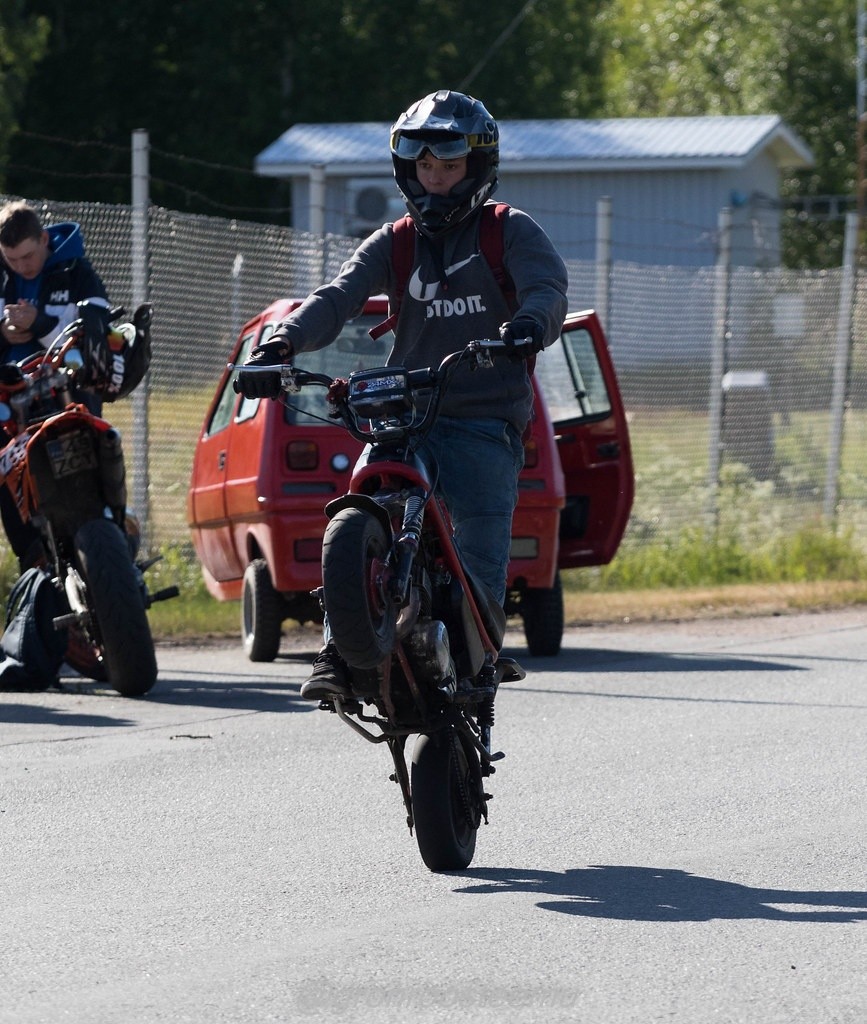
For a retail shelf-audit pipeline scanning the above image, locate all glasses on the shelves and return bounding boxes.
[395,130,471,160]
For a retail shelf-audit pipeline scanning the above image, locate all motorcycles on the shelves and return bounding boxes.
[187,292,636,663]
[0,304,181,699]
[221,333,540,875]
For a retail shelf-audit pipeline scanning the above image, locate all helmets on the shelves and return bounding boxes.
[104,302,155,403]
[389,90,500,239]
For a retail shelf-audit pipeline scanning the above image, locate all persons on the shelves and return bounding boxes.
[240,91,569,699]
[0,202,106,575]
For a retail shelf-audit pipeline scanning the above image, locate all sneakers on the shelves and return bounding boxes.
[300,644,356,700]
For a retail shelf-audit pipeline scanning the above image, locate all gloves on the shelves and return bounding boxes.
[237,341,292,400]
[498,317,543,362]
[0,359,23,385]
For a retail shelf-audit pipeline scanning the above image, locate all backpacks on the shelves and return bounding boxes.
[0,566,70,691]
